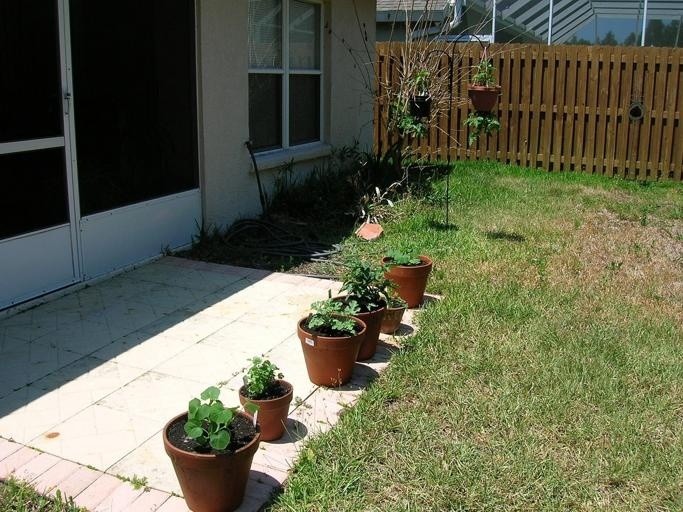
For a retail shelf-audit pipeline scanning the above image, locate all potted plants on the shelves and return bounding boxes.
[407,46,502,117]
[297,296,366,387]
[163,387,261,510]
[380,241,434,309]
[326,259,388,363]
[380,281,406,334]
[239,357,293,442]
